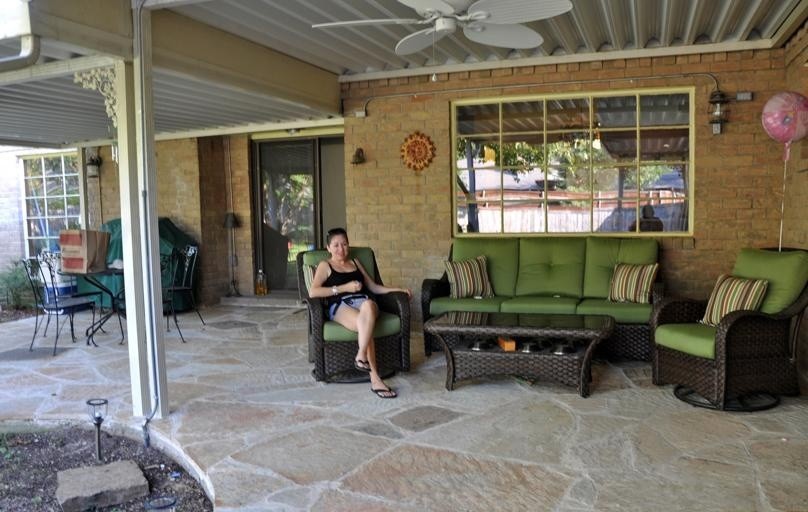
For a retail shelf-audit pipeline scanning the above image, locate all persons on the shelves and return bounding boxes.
[308,228,412,398]
[628,205,663,232]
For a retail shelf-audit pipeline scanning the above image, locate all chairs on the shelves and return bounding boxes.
[21,243,207,354]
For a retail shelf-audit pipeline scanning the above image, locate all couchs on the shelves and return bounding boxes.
[295,247,413,385]
[648,246,807,413]
[422,236,663,360]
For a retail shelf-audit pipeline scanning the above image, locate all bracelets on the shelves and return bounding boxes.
[332,285,338,296]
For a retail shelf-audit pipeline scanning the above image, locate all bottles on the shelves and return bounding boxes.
[255,269,268,297]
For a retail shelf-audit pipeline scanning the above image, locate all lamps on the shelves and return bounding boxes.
[704,74,734,135]
[222,212,243,296]
[86,155,102,178]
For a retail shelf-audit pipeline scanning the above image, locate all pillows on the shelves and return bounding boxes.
[608,262,660,306]
[441,255,496,299]
[701,272,769,328]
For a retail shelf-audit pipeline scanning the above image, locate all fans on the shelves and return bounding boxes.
[308,1,575,57]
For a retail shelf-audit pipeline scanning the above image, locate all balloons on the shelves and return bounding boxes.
[761,91,808,144]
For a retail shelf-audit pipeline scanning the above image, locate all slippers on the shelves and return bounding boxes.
[354,359,371,372]
[370,388,397,398]
